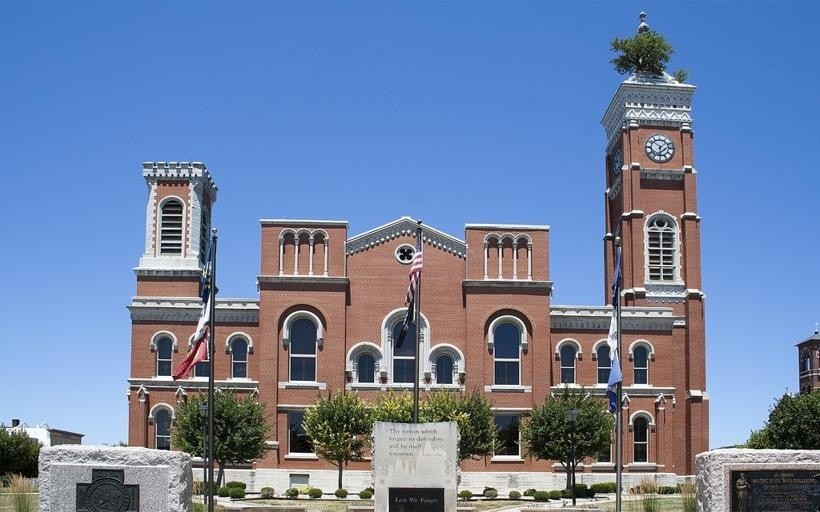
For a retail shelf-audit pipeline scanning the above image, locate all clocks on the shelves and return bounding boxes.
[644,134,675,163]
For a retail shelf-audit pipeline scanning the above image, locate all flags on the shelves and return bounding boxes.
[395,225,424,349]
[606,245,625,429]
[172,242,219,381]
